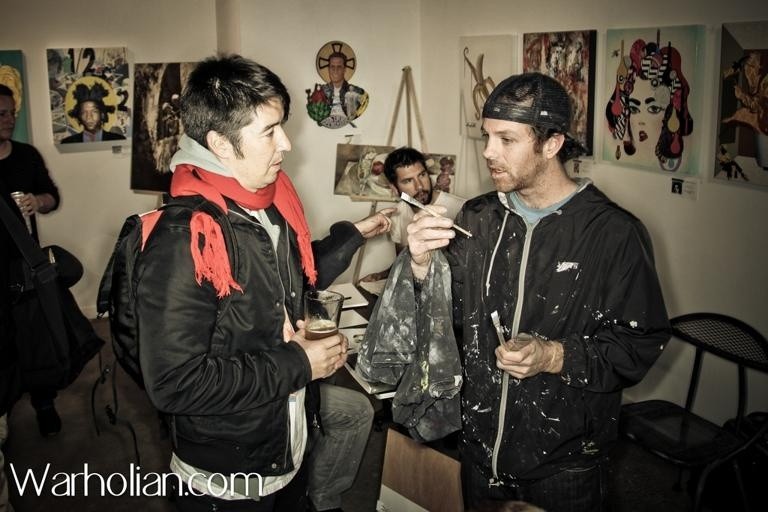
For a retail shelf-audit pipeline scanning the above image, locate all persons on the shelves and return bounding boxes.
[1,84,64,436]
[355,147,470,286]
[479,500,544,511]
[405,71,672,511]
[61,80,126,144]
[137,51,347,511]
[304,206,395,509]
[318,46,360,113]
[602,42,692,172]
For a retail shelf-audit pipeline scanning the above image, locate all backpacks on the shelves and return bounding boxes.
[95,193,239,390]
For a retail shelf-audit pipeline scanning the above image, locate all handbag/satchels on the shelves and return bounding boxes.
[8,278,105,391]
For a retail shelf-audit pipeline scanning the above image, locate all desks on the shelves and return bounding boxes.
[331,283,466,432]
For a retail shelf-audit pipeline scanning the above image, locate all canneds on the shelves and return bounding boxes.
[10,191,33,235]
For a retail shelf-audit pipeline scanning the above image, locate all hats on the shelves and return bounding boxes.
[482,73,589,161]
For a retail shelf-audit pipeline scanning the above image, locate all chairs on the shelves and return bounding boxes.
[623,312,768,511]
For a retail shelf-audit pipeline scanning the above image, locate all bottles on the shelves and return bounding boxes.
[11,191,33,236]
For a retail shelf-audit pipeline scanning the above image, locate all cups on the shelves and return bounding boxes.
[303,287,343,379]
